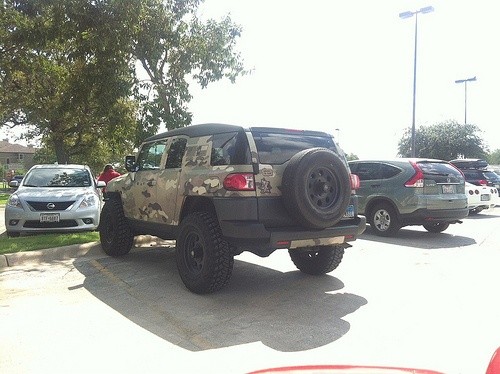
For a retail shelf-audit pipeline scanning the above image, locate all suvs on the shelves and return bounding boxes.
[99,122,365,296]
[347,158,470,237]
[448,159,500,198]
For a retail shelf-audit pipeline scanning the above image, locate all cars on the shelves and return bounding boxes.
[96,163,128,201]
[11,175,24,185]
[464,181,499,215]
[5,161,107,237]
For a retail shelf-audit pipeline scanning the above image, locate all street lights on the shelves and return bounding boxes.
[400,5,435,158]
[455,75,480,125]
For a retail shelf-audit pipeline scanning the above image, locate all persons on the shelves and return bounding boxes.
[97,164,122,203]
